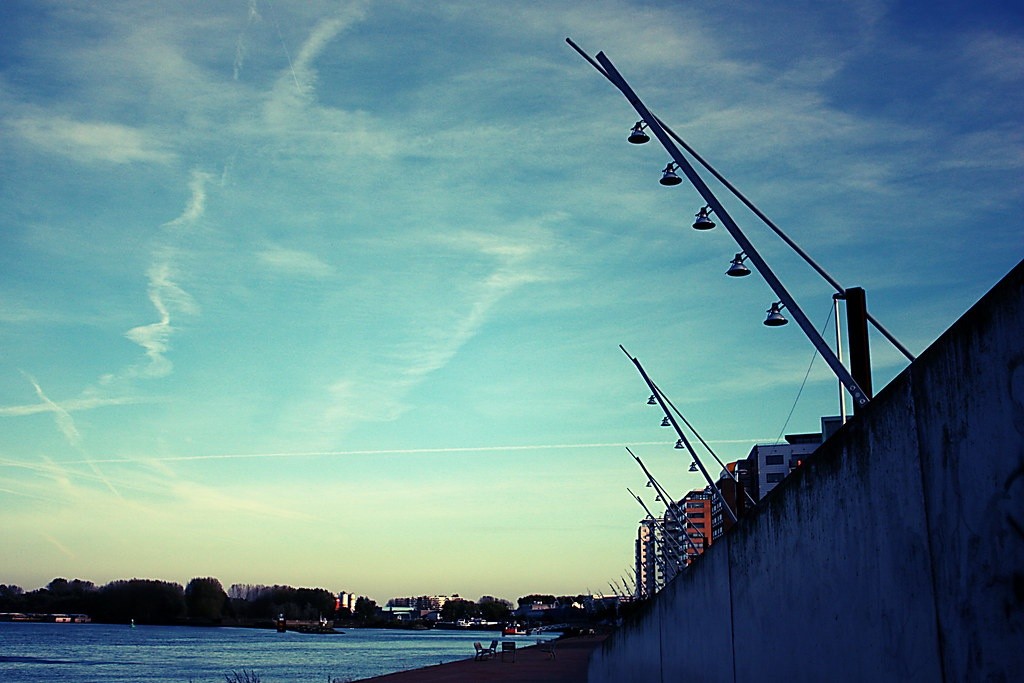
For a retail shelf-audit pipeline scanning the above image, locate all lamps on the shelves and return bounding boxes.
[628,120,651,144]
[647,395,657,404]
[661,416,671,426]
[689,462,698,471]
[674,439,684,448]
[660,161,682,186]
[764,300,788,326]
[725,251,751,277]
[692,204,716,230]
[646,481,652,487]
[655,495,661,501]
[704,485,711,491]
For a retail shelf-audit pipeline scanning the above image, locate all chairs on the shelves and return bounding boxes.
[474,640,498,661]
[537,639,560,661]
[501,642,517,663]
